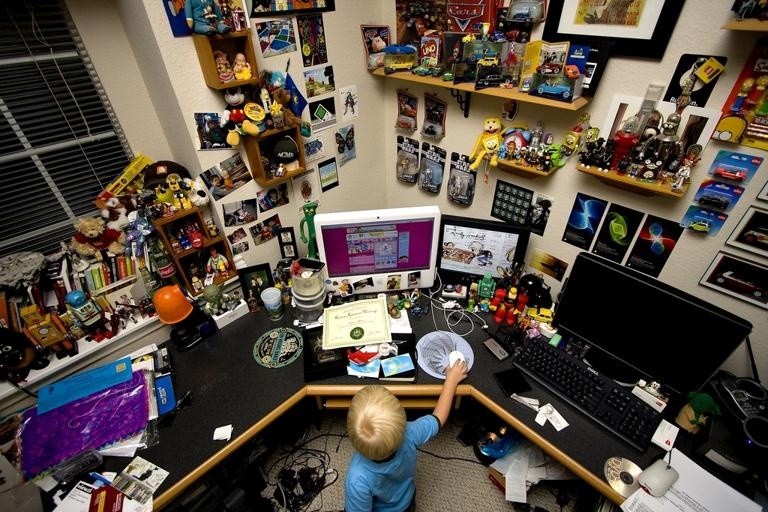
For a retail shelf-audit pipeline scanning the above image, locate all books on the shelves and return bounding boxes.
[7,281,40,328]
[72,252,136,293]
[377,344,417,383]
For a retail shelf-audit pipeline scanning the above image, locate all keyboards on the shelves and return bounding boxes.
[513,336,663,453]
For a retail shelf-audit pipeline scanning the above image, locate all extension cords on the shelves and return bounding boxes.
[442,285,467,299]
[273,475,298,508]
[273,467,319,512]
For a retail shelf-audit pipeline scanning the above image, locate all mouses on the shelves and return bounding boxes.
[448,351,467,369]
[638,459,679,497]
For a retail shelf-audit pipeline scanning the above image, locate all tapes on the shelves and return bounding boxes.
[378,342,391,356]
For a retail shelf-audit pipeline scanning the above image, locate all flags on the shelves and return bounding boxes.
[284,74,307,116]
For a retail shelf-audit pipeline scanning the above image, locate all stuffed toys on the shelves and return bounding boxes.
[69,218,126,257]
[0,328,50,384]
[200,283,242,317]
[274,89,302,126]
[101,198,128,231]
[220,87,259,145]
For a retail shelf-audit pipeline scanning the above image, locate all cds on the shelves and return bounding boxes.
[604,456,643,500]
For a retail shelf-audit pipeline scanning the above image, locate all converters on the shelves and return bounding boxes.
[277,468,296,483]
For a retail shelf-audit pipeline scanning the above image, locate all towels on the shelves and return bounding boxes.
[212,423,234,442]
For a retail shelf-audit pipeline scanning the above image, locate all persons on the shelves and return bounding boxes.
[443,242,493,268]
[250,276,264,290]
[234,209,255,222]
[64,291,112,342]
[139,294,155,318]
[20,305,79,359]
[223,172,237,191]
[185,0,230,34]
[210,175,228,201]
[267,188,279,208]
[115,295,138,330]
[257,88,278,127]
[643,380,663,398]
[344,359,469,512]
[199,113,218,143]
[525,198,552,231]
[213,51,253,82]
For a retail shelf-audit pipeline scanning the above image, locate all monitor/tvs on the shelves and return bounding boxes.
[436,214,531,286]
[314,206,441,297]
[553,252,755,403]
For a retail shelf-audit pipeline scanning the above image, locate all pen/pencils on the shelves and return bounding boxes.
[391,341,407,344]
[176,390,192,408]
[90,471,132,502]
[111,467,153,504]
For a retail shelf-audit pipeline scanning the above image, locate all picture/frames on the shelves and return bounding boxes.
[541,1,685,62]
[698,251,768,314]
[724,205,768,260]
[249,0,336,18]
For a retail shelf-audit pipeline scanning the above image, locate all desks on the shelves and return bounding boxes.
[39,290,768,512]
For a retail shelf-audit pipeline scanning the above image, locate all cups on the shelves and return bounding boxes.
[259,287,284,320]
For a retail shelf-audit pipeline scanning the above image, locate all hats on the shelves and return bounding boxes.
[272,138,298,164]
[142,158,191,189]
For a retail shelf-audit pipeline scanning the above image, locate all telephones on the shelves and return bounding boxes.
[709,363,768,433]
